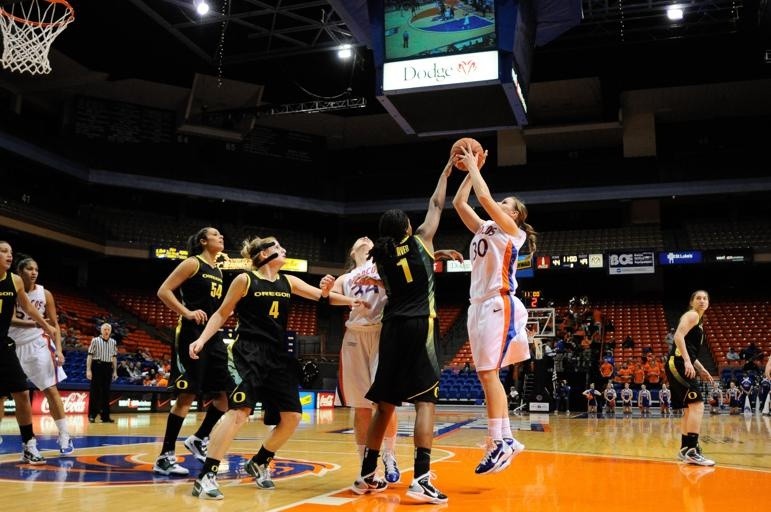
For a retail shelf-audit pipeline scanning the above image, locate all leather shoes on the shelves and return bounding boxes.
[89,415,96,423]
[100,417,114,423]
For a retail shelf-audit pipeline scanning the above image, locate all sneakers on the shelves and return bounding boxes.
[473,439,512,474]
[57,433,75,455]
[245,457,276,490]
[185,434,210,464]
[153,452,189,475]
[684,447,715,465]
[192,472,225,499]
[677,446,688,461]
[495,436,524,472]
[351,449,448,505]
[23,438,47,465]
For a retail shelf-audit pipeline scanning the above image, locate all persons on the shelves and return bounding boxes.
[348,148,463,506]
[546,296,601,366]
[153,225,229,478]
[91,312,132,344]
[319,231,465,484]
[117,347,170,387]
[450,140,540,475]
[605,321,616,349]
[710,344,771,415]
[665,289,721,467]
[555,381,570,412]
[644,344,652,353]
[86,323,117,422]
[582,351,682,414]
[190,233,366,502]
[385,1,494,52]
[8,257,78,455]
[622,336,634,348]
[666,328,676,349]
[58,309,82,346]
[0,240,61,467]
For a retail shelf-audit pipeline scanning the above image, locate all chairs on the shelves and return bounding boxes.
[438,303,771,403]
[54,295,315,390]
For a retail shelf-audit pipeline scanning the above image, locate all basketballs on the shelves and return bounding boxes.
[451,138,483,171]
